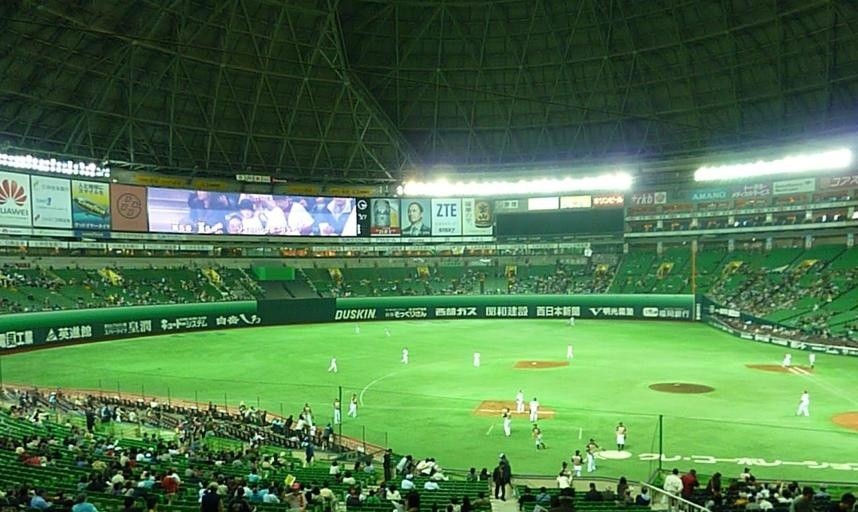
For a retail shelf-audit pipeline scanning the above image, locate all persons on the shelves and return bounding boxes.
[402,202,430,236]
[0,250,857,510]
[187,193,359,236]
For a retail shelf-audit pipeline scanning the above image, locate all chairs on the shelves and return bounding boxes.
[0,407,668,512]
[0,243,858,342]
